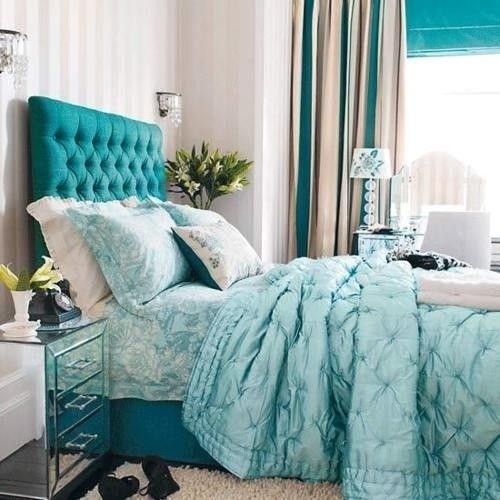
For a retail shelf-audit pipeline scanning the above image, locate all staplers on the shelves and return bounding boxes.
[378,229,394,235]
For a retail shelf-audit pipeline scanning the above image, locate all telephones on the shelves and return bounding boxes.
[28,278,81,324]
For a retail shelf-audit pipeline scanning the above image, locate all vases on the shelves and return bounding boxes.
[10,290,33,321]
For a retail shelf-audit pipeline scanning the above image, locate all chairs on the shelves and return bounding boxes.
[419,211,492,273]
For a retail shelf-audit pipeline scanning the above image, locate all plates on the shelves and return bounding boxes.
[0,321,41,337]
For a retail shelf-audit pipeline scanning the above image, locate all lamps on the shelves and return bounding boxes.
[348,146,393,234]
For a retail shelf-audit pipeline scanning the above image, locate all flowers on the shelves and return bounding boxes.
[1,254,64,296]
[26,194,266,318]
[164,139,255,209]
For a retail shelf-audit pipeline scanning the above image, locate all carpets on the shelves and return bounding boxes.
[79,461,342,500]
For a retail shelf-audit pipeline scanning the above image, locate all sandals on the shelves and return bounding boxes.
[98,472,139,500]
[139,454,179,500]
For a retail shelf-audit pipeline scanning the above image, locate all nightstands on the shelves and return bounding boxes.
[0,320,123,500]
[26,95,500,484]
